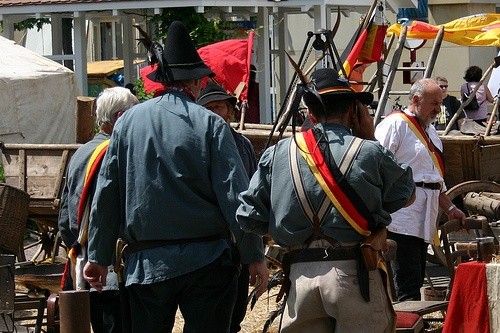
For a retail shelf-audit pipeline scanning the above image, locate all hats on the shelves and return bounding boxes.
[146,21,216,83]
[195,80,237,111]
[303,68,374,106]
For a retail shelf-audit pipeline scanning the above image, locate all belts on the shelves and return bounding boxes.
[415,182,441,190]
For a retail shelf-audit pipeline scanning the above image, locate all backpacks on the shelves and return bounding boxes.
[463,83,487,111]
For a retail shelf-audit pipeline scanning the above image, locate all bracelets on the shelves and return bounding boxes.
[359,235,376,254]
[444,200,460,218]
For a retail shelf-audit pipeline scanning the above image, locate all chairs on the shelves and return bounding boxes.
[392,300,449,313]
[441,217,498,277]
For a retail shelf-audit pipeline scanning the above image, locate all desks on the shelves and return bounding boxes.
[441,260,500,333]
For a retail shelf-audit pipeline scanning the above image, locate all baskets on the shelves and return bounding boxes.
[1,181,31,254]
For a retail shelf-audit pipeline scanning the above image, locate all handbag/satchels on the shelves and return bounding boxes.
[359,226,391,271]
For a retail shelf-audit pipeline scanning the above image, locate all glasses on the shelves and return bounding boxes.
[439,85,448,89]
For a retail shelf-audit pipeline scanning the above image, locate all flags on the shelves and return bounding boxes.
[138,31,251,104]
[387,11,500,48]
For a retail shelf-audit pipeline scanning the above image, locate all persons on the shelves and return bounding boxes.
[458,65,492,125]
[375,79,466,306]
[85,21,267,333]
[241,66,395,332]
[434,76,461,130]
[190,80,259,178]
[59,86,141,333]
